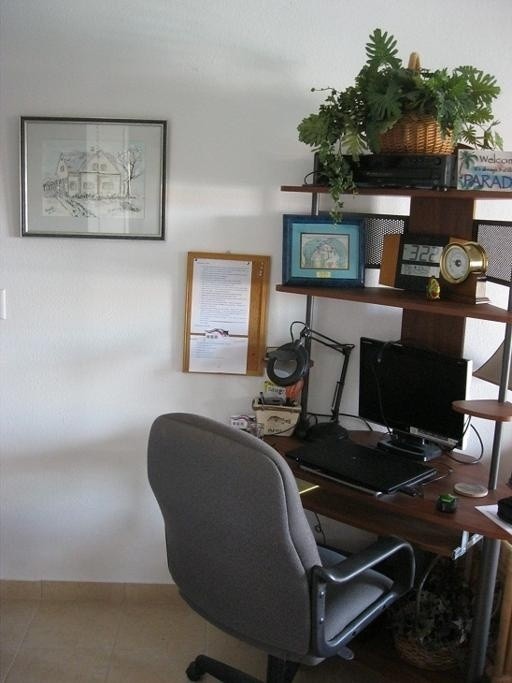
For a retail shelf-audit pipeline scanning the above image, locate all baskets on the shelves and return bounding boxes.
[365,53,458,155]
[394,554,472,672]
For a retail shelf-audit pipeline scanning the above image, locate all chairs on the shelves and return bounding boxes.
[145,411,419,681]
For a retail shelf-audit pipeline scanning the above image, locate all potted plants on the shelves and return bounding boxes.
[296,28,505,226]
[389,581,477,671]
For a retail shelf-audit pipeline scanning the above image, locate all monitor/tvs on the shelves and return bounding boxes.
[358,335,474,462]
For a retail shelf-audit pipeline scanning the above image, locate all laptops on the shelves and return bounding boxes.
[285,437,438,502]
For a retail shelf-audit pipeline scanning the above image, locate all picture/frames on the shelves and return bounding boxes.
[281,213,367,290]
[18,115,166,241]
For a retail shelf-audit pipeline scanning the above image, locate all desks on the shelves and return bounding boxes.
[259,429,511,681]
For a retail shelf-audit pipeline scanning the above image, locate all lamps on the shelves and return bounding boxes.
[264,319,355,441]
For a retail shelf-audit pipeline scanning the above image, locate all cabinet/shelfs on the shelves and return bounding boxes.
[275,184,511,327]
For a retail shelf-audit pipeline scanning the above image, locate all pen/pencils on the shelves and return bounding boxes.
[259,392,266,405]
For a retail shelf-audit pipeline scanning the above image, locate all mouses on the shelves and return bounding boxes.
[433,491,463,514]
